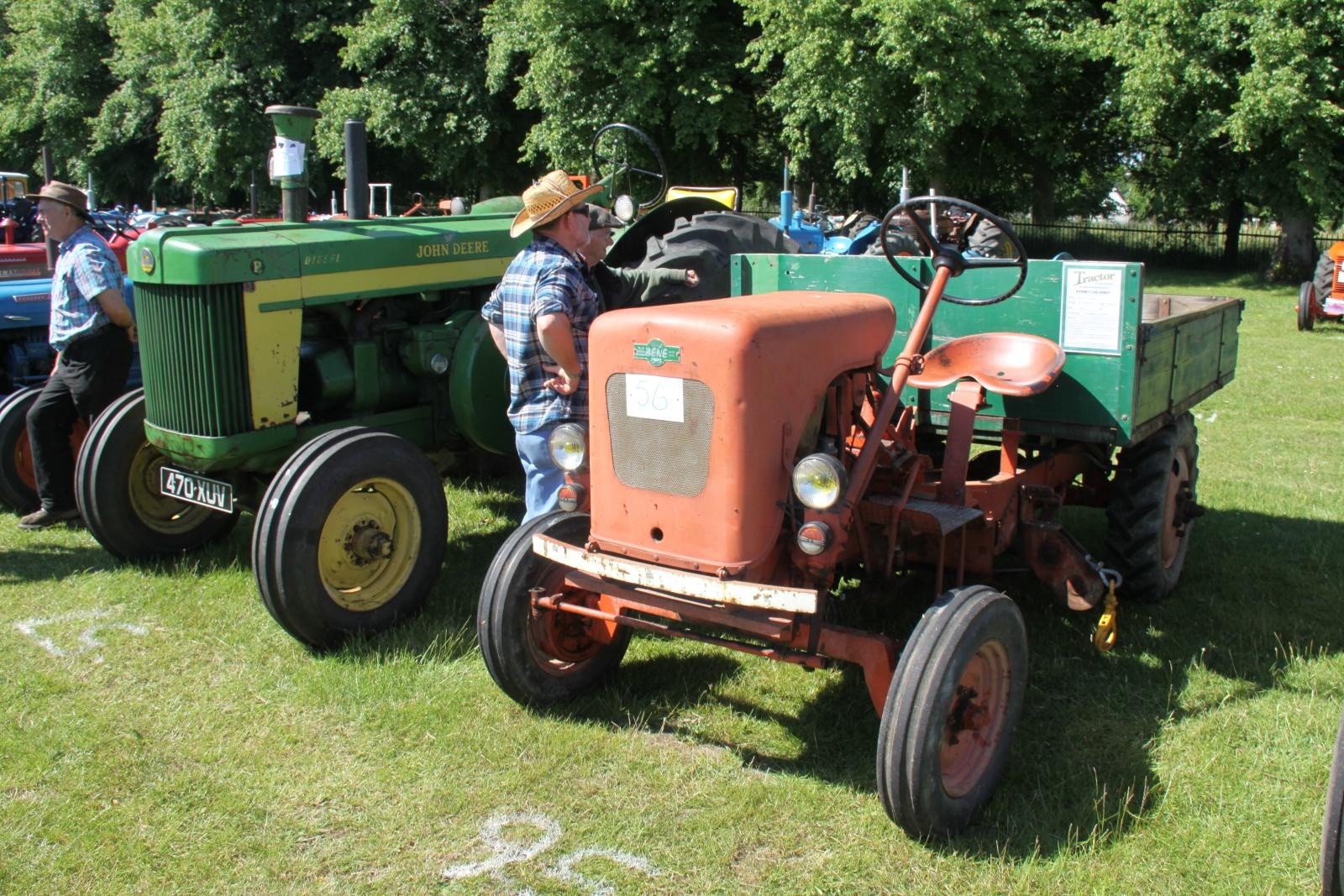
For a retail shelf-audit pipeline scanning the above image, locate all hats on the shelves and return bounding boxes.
[583,203,623,230]
[24,179,97,226]
[509,169,606,237]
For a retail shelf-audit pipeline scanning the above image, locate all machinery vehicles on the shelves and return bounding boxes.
[53,101,804,662]
[0,161,463,519]
[475,190,1258,853]
[1293,219,1344,333]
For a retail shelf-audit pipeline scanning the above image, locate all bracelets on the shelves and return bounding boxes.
[566,362,584,379]
[126,320,136,330]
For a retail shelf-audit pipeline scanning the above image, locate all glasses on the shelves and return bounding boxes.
[570,204,591,216]
[596,231,616,239]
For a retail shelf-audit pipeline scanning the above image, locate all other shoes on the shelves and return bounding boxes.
[21,504,78,526]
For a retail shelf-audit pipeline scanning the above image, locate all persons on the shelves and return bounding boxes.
[19,181,138,529]
[482,171,605,526]
[573,203,699,313]
